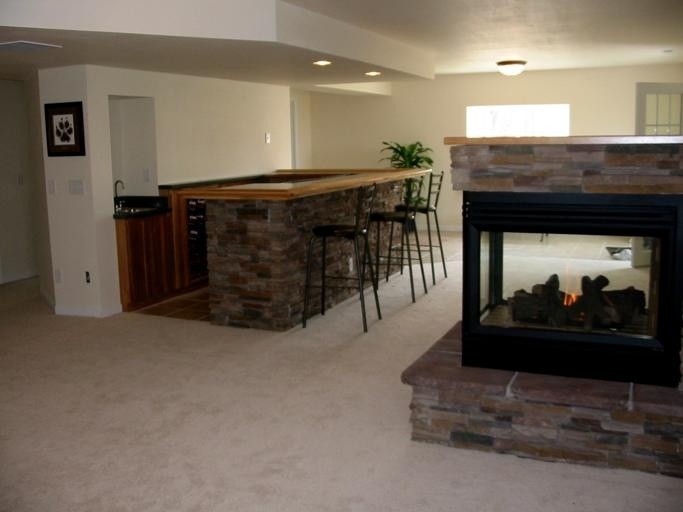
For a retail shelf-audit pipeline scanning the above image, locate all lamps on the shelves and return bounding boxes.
[493,58,529,78]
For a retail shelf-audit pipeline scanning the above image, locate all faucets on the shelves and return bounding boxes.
[115,179,125,196]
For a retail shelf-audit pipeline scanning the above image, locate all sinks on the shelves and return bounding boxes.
[119,208,151,214]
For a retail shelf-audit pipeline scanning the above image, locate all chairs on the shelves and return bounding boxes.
[395,169,447,282]
[299,178,382,333]
[358,174,428,304]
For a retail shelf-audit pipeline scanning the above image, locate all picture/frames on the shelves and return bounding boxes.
[42,99,86,161]
